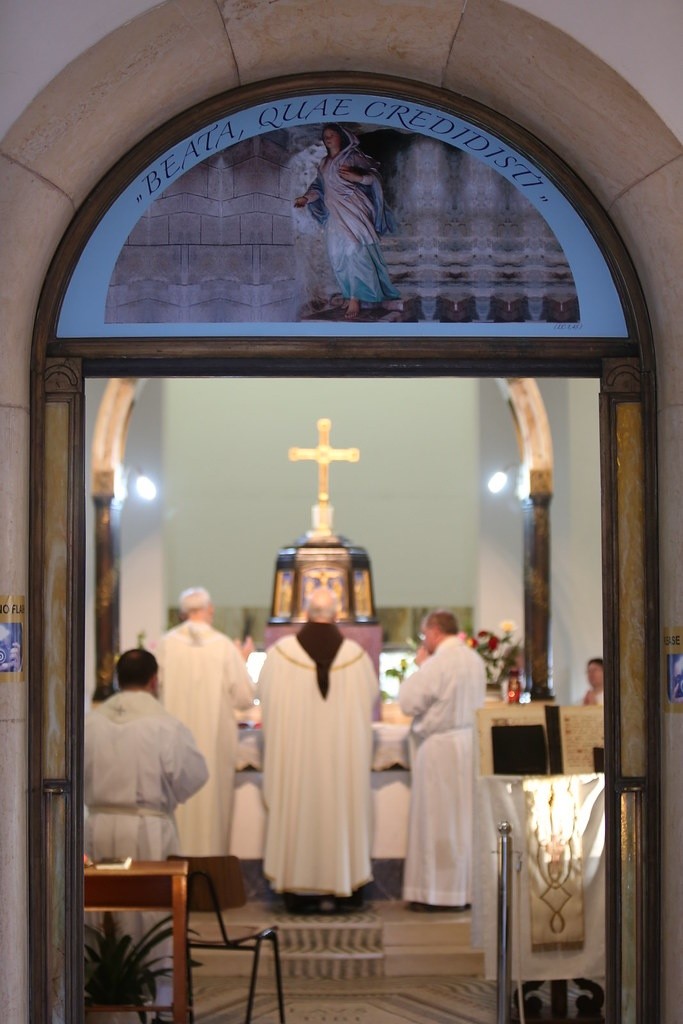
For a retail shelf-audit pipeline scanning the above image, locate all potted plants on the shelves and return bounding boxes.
[83,913,204,1024]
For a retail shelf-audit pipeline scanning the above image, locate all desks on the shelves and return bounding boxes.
[84,860,193,1024]
[228,721,463,860]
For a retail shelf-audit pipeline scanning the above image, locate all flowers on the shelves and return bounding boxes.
[458,620,523,686]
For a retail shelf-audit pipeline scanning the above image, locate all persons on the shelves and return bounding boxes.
[257,588,379,916]
[153,587,257,857]
[293,122,401,318]
[399,613,487,914]
[584,659,604,705]
[84,649,210,1024]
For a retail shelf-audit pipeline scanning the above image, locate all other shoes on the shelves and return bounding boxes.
[290,900,317,915]
[334,899,364,910]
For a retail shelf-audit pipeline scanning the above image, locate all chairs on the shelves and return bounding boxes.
[166,854,286,1024]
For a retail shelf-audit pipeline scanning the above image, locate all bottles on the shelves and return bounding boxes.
[509,670,520,703]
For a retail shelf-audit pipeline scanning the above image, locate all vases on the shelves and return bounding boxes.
[507,670,521,705]
